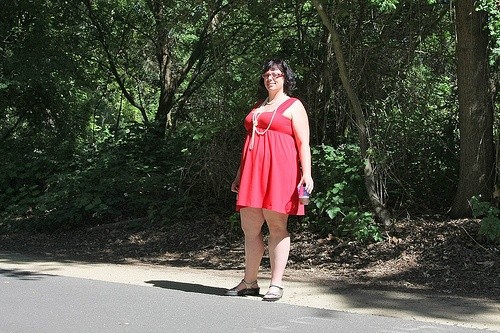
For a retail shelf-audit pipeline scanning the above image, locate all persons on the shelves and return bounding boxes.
[223,58,314,301]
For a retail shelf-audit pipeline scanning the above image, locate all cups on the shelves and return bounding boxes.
[297,187,310,205]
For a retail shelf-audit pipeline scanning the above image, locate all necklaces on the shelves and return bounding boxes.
[248,91,288,150]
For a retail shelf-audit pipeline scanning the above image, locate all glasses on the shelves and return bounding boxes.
[262,73,286,79]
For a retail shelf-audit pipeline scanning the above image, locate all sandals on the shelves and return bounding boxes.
[226,279,259,296]
[262,285,284,301]
